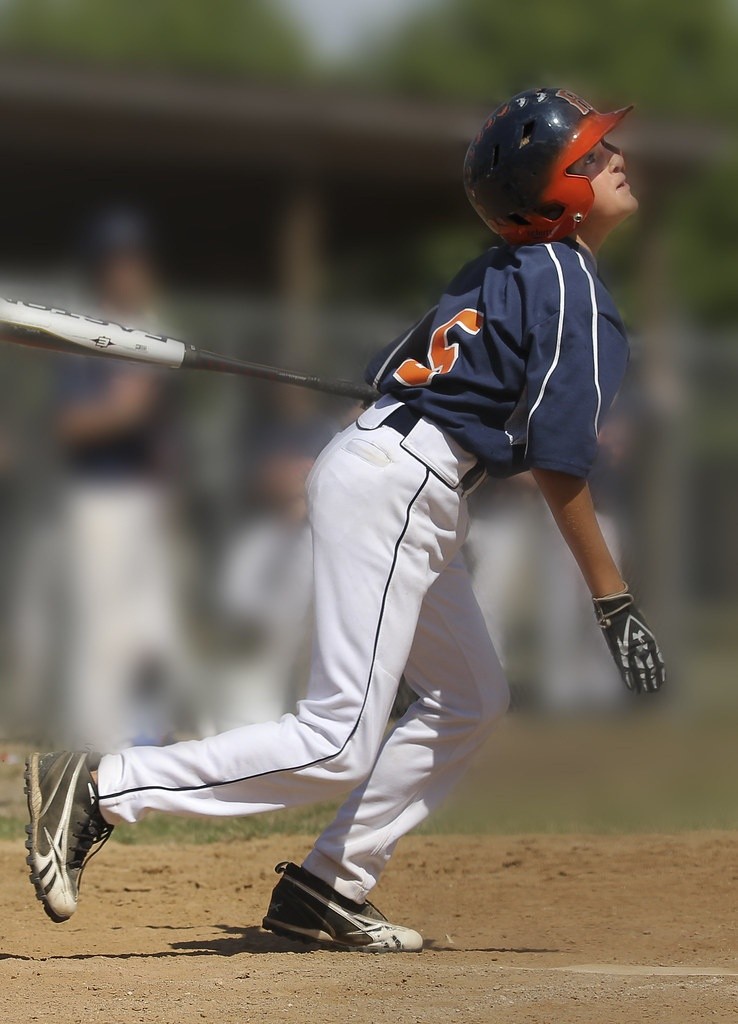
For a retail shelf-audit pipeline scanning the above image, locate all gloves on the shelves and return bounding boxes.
[587,582,669,697]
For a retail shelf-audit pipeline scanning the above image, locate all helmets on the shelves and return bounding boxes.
[463,86,634,248]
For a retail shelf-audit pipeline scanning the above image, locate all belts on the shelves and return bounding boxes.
[384,400,483,488]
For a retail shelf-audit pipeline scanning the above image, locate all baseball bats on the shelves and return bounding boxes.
[1,281,385,405]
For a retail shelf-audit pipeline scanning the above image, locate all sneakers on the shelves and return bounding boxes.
[262,863,423,953]
[24,750,114,924]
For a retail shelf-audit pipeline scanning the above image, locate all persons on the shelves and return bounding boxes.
[28,84,670,954]
[4,193,738,767]
